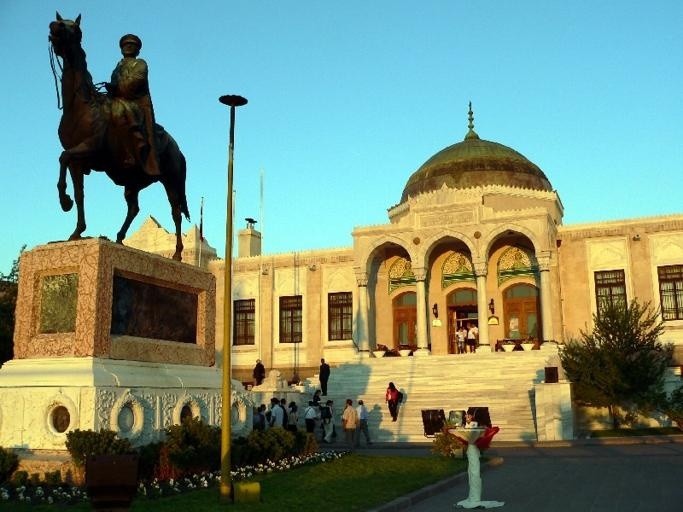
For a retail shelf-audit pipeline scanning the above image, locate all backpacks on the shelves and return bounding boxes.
[321,407,332,419]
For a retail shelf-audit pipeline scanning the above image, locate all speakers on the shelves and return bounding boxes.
[545,367,558,383]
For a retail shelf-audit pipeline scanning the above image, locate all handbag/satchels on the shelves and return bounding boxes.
[398,392,403,402]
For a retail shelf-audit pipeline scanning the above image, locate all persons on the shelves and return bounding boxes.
[454,322,479,354]
[463,414,479,429]
[252,389,375,449]
[253,359,264,387]
[385,382,400,422]
[106,34,155,165]
[318,358,330,396]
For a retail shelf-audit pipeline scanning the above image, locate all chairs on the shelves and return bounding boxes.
[420,407,499,459]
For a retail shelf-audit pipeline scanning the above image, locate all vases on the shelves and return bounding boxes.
[398,349,411,356]
[372,350,386,358]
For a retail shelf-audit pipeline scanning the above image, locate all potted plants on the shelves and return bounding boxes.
[500,340,515,352]
[520,339,535,353]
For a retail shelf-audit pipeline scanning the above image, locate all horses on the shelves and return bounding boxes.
[48,10,190,263]
